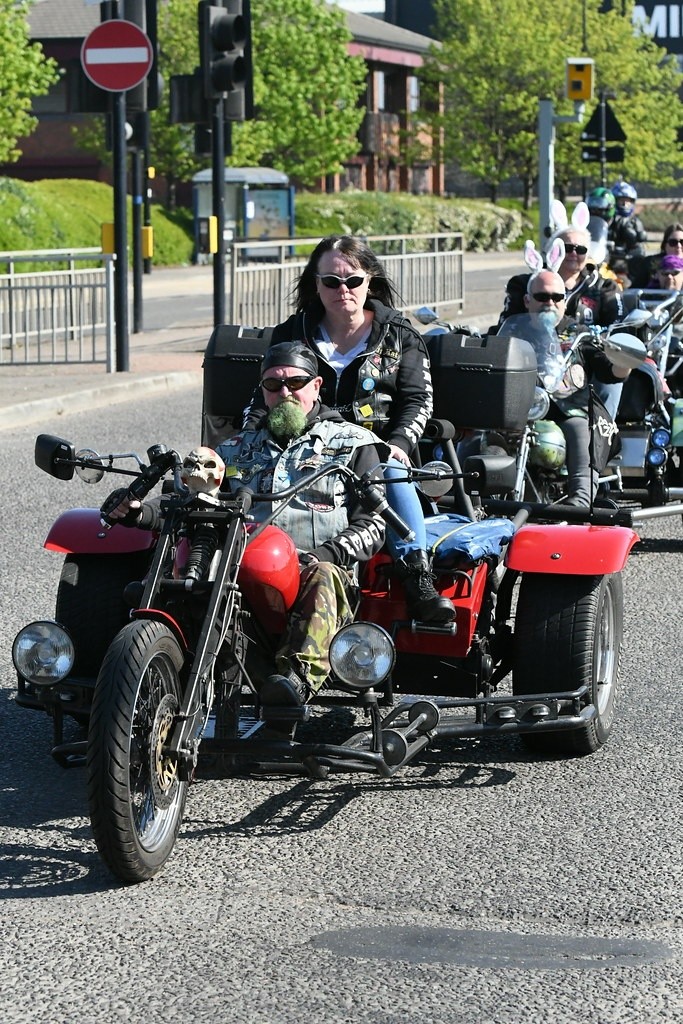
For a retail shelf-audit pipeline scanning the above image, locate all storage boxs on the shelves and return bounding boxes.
[427,333,527,430]
[201,324,278,418]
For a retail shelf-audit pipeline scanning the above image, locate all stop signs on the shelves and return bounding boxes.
[80,21,153,94]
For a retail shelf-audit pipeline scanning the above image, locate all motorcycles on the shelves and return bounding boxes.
[7,426,651,885]
[208,267,683,576]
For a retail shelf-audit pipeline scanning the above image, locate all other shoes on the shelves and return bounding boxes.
[563,498,584,525]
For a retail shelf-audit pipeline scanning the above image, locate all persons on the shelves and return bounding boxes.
[632,222,683,289]
[550,230,624,327]
[244,235,456,627]
[482,272,632,507]
[586,181,647,251]
[101,342,388,707]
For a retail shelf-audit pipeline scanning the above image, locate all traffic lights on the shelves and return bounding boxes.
[197,1,251,106]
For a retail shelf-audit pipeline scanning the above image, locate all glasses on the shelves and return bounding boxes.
[529,292,566,302]
[316,274,370,289]
[565,243,588,255]
[665,238,683,247]
[660,269,681,276]
[259,375,316,392]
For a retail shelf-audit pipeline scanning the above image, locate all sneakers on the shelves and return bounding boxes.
[260,669,317,725]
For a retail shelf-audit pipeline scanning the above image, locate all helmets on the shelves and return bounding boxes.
[612,181,638,217]
[585,187,616,223]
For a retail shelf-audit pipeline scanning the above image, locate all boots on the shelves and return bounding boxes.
[397,548,456,623]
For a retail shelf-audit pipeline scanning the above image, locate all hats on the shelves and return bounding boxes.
[657,254,683,272]
[260,341,318,377]
